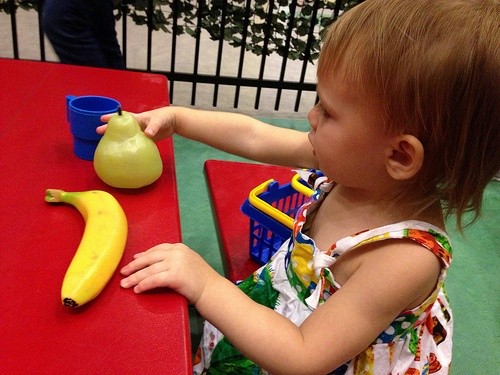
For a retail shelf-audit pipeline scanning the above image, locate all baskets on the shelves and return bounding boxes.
[240,169,320,266]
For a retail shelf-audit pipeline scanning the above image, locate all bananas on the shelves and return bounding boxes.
[43,188,128,310]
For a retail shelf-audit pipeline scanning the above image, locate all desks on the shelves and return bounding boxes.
[0,56,193,375]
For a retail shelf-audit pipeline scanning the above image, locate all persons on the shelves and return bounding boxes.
[94,0,499,374]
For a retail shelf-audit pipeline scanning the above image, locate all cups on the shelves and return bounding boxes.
[66,95,121,161]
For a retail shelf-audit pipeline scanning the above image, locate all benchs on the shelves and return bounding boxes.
[201,159,317,287]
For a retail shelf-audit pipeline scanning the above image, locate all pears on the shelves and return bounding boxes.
[92,106,163,190]
